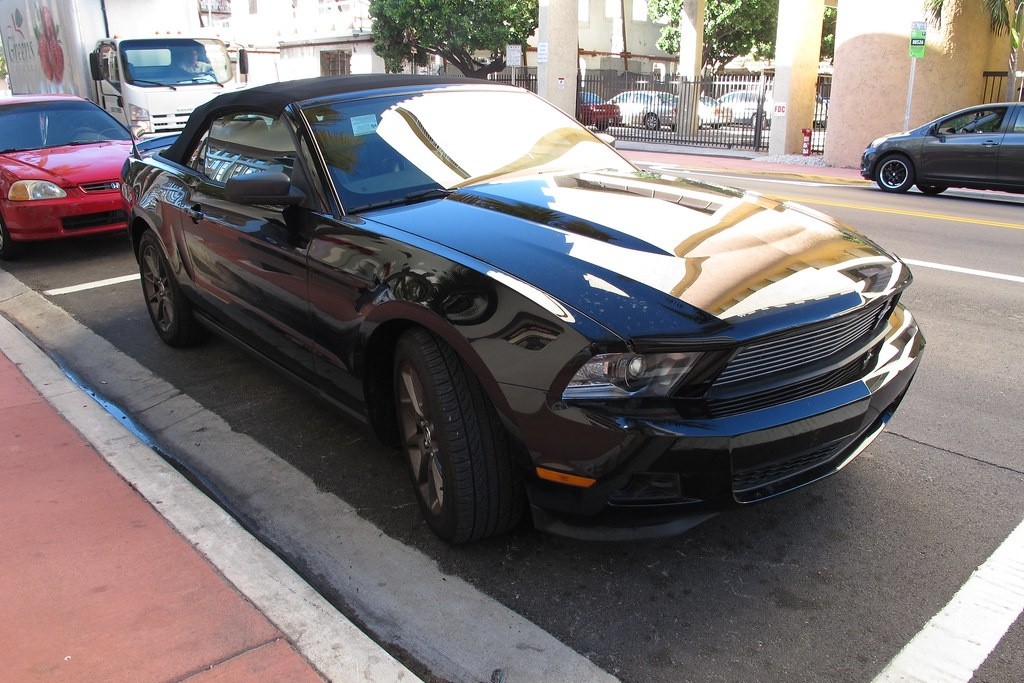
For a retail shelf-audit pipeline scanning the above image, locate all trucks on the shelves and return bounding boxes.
[0,0,249,139]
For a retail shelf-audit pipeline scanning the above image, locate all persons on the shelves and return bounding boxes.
[179,50,210,73]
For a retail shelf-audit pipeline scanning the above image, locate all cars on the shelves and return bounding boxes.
[813,92,829,128]
[0,94,140,261]
[697,96,734,131]
[579,92,623,132]
[860,102,1024,196]
[606,90,678,131]
[119,76,926,550]
[716,91,772,130]
